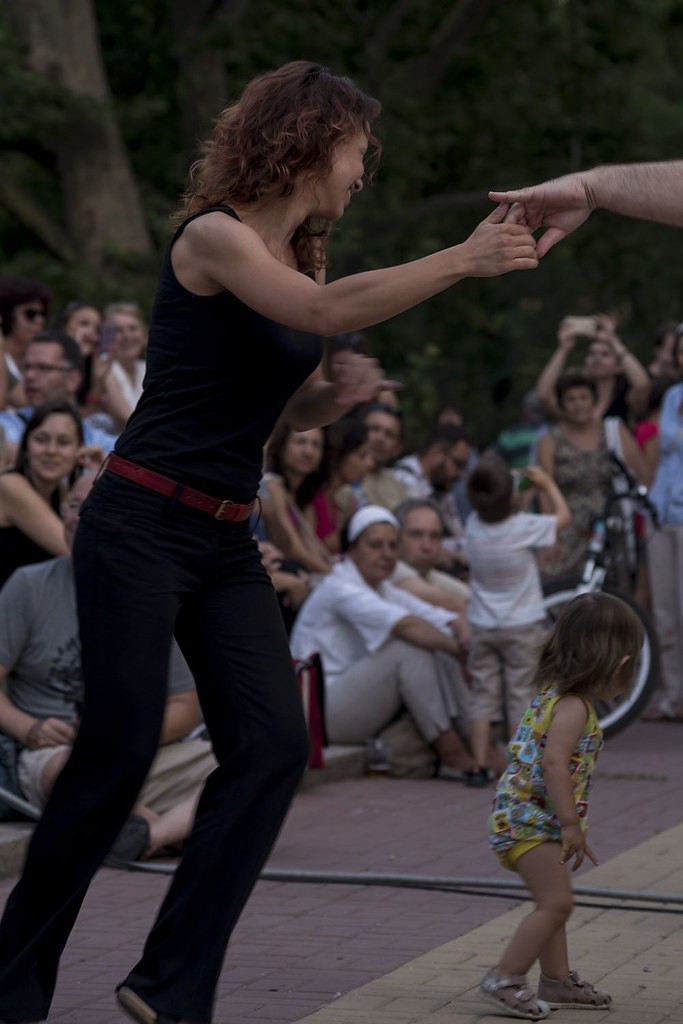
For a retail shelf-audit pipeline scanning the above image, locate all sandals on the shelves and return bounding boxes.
[538,970,613,1009]
[477,967,550,1019]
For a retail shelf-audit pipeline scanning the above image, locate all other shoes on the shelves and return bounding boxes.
[117,984,177,1024]
[108,813,150,861]
[465,766,488,785]
[641,699,683,720]
[438,753,503,782]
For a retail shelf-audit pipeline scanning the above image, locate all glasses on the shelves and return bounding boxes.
[23,309,48,319]
[18,362,71,372]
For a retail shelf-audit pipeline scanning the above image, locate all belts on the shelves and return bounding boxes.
[107,452,256,522]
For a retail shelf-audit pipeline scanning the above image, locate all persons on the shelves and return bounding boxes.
[477,590,645,1019]
[0,279,683,858]
[0,60,539,1022]
[487,160,683,259]
[287,501,467,782]
[458,460,571,789]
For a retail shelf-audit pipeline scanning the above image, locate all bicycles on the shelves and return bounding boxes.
[542,446,664,736]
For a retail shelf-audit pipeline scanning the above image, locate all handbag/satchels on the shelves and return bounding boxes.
[292,653,329,769]
[367,714,439,779]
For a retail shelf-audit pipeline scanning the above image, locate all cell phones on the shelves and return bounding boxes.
[567,316,596,336]
[99,324,118,359]
